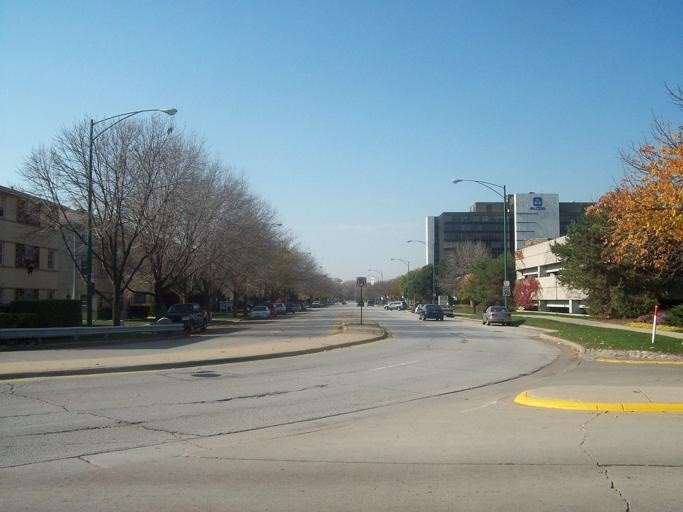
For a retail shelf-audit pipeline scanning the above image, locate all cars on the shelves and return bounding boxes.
[246,299,325,321]
[357,300,374,307]
[481,305,510,326]
[159,302,209,335]
[411,302,453,321]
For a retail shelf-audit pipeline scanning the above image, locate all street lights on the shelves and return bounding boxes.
[86,109,177,326]
[406,239,435,304]
[368,257,409,305]
[451,177,509,308]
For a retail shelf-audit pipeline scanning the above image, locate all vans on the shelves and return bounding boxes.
[383,301,406,311]
